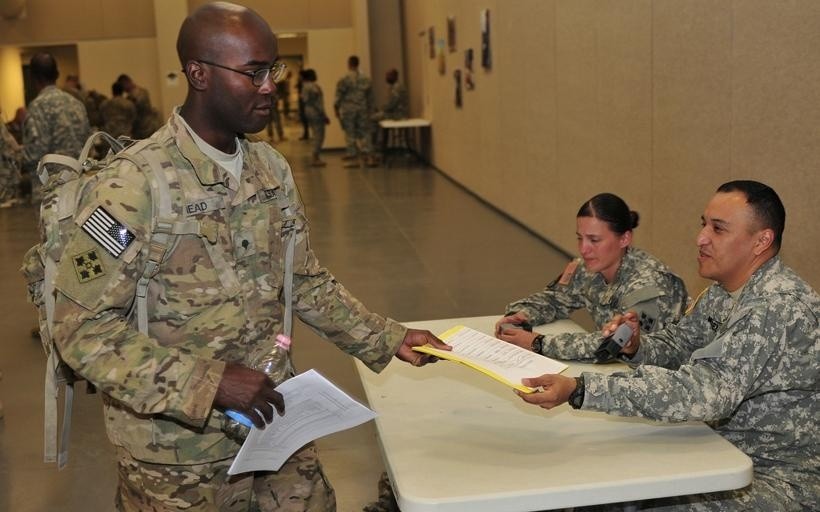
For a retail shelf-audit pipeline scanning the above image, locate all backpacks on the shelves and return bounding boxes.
[18,132,295,383]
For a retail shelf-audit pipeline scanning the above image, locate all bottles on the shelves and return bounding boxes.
[219,333,291,444]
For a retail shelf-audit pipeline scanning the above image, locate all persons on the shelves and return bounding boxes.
[495,193,688,363]
[18,0,453,512]
[512,180,819,512]
[0,52,153,208]
[267,56,409,167]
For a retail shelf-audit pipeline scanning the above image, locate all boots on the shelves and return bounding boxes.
[311,149,378,168]
[31,328,40,337]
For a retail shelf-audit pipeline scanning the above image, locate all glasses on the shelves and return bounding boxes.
[197,60,287,86]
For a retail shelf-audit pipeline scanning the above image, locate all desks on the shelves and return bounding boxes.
[377,118,431,164]
[352,314,753,512]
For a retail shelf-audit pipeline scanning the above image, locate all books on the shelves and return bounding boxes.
[412,324,569,394]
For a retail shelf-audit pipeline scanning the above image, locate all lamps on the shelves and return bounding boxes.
[0,0,27,32]
